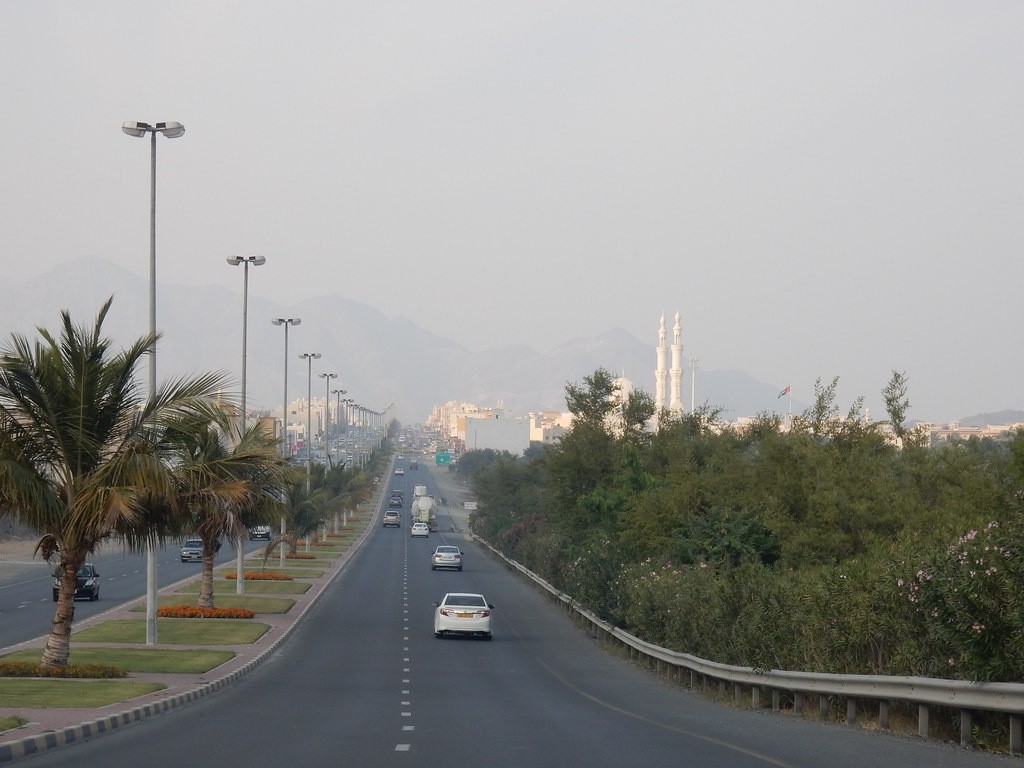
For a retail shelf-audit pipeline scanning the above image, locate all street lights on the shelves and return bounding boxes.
[272,318,303,567]
[319,373,337,542]
[122,119,188,648]
[228,255,267,600]
[299,353,322,553]
[331,388,395,532]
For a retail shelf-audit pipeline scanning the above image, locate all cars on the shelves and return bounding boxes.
[430,545,464,572]
[383,511,402,528]
[390,489,405,497]
[51,561,101,602]
[409,522,430,538]
[433,593,495,640]
[398,429,418,471]
[248,524,273,541]
[389,495,402,508]
[395,468,404,476]
[289,427,382,468]
[179,539,205,562]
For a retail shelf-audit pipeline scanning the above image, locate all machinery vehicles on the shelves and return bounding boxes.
[409,494,439,531]
